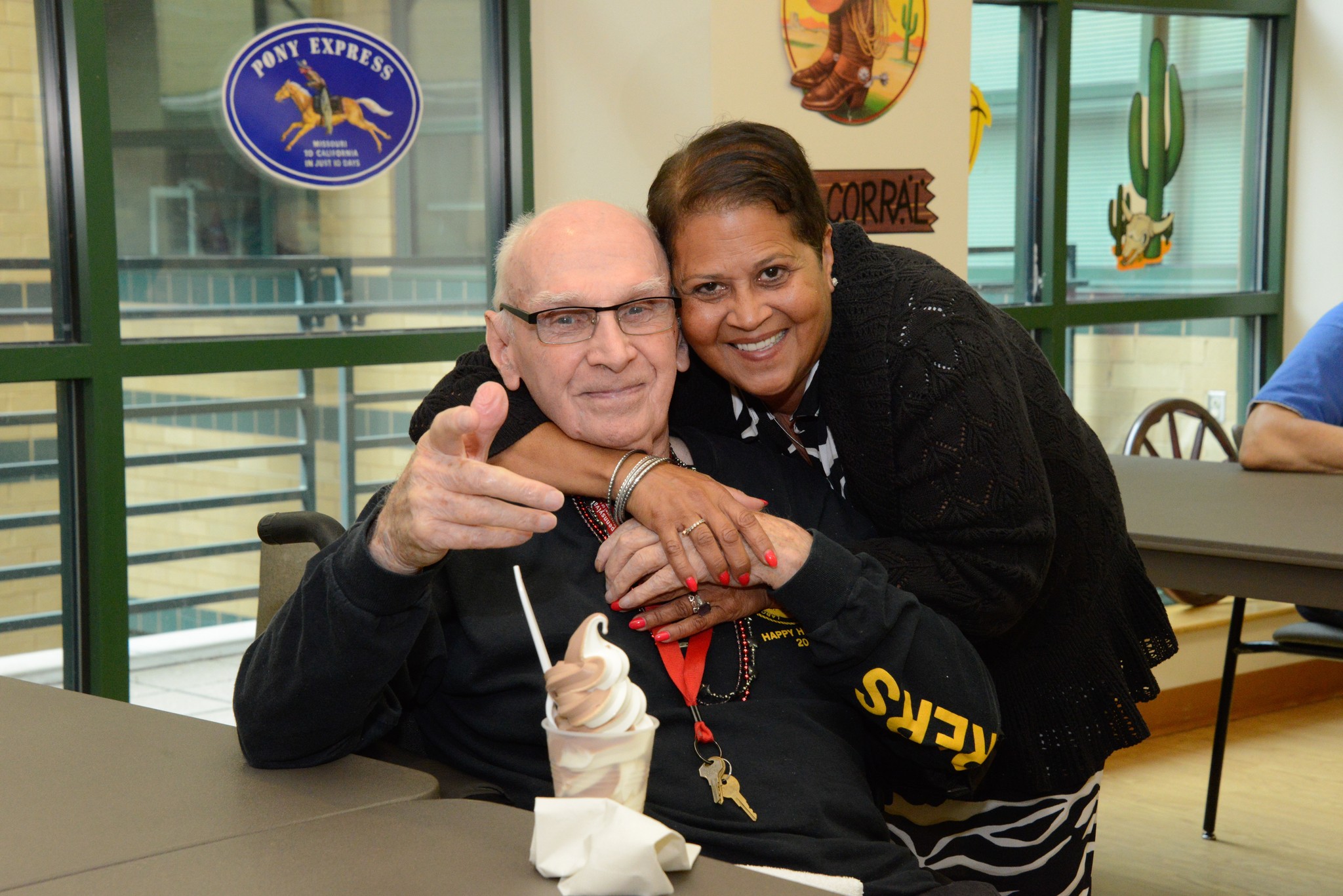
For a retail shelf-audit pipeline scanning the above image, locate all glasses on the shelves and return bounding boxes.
[498,296,686,345]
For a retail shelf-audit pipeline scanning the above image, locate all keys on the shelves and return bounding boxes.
[699,757,758,821]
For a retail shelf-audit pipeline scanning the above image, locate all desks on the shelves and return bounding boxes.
[0,673,851,896]
[1108,450,1343,608]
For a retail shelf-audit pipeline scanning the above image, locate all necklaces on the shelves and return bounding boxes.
[571,444,757,704]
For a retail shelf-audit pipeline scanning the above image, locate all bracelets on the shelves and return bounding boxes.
[607,450,672,525]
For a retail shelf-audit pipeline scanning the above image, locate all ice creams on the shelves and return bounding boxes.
[543,613,653,815]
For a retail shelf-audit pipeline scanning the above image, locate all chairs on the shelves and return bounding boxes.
[1120,399,1343,840]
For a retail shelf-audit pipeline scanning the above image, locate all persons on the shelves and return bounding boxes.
[1237,300,1342,627]
[410,123,1178,896]
[236,202,1000,896]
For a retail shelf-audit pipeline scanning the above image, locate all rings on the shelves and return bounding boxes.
[682,519,706,535]
[688,592,712,616]
